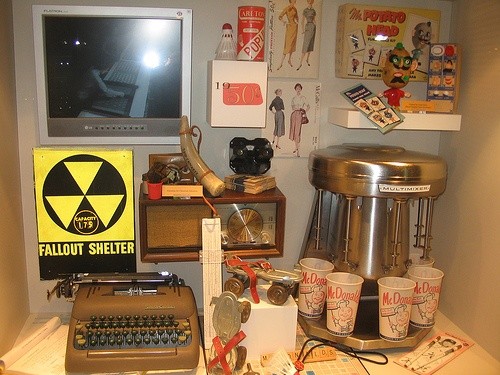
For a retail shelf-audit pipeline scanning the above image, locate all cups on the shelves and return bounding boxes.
[325,272,364,338]
[376,276,417,343]
[298,257,334,318]
[408,253,435,269]
[146,181,163,200]
[406,266,445,329]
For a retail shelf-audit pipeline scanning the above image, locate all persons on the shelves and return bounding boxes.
[73,57,130,107]
[378,42,418,112]
[99,26,123,63]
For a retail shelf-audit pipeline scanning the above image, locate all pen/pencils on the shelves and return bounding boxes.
[404,336,442,368]
[412,344,462,371]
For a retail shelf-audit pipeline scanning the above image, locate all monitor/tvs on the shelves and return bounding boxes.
[31,4,191,145]
